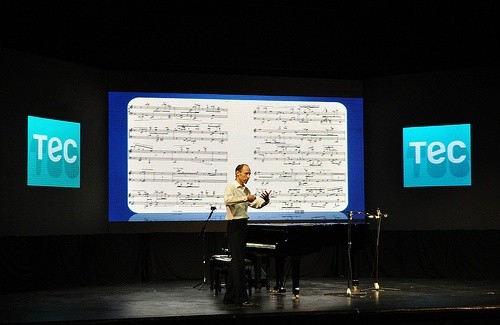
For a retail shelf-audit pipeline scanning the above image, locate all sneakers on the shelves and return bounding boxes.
[240,297,256,307]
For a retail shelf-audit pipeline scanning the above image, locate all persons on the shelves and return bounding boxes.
[223,163,272,307]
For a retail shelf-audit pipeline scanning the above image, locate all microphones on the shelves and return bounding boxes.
[346,211,362,215]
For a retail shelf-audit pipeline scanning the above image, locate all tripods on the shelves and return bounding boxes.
[324,214,367,299]
[360,208,400,292]
[193,207,217,290]
